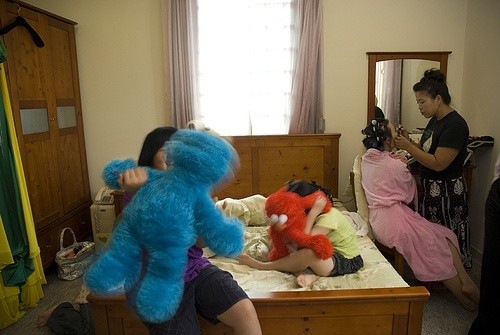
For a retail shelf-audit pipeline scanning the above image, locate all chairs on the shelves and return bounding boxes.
[350,172,405,276]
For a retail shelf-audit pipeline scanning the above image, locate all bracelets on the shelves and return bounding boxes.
[410,137,411,142]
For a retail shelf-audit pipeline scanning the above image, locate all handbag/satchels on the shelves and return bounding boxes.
[56,227,95,280]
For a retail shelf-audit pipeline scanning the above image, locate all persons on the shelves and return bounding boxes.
[361,118,481,311]
[117,126,262,335]
[393,68,473,273]
[237,179,364,287]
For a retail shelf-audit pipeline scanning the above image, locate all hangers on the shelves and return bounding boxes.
[0,4,46,48]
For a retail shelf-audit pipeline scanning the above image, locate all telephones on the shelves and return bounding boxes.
[93,186,115,204]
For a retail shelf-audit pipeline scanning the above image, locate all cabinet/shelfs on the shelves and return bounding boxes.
[0,0,93,273]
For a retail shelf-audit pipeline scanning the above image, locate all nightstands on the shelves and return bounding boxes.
[90,204,116,256]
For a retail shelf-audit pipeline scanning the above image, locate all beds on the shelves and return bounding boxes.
[85,137,431,335]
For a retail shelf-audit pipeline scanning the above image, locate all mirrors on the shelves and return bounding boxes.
[365,51,453,134]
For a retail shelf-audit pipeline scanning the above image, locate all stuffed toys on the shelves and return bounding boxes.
[85,121,245,322]
[216,193,268,227]
[264,186,335,261]
